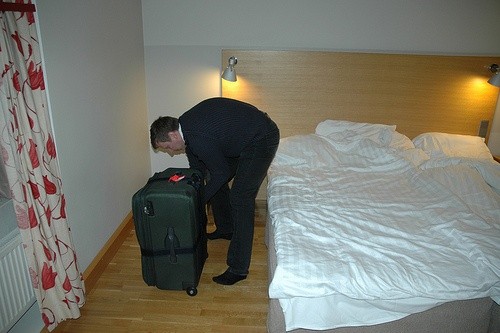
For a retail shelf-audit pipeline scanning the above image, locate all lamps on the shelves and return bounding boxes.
[486,64,500,87]
[221,56,238,81]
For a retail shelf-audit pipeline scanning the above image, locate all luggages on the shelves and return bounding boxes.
[132,167,209,296]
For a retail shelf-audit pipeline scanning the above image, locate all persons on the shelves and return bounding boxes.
[149,97,281,285]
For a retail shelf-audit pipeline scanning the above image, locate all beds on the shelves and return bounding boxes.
[265,120,500,333]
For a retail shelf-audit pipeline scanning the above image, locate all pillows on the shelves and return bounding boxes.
[413,132,494,160]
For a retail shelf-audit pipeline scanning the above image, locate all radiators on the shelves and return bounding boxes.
[0,229,34,333]
